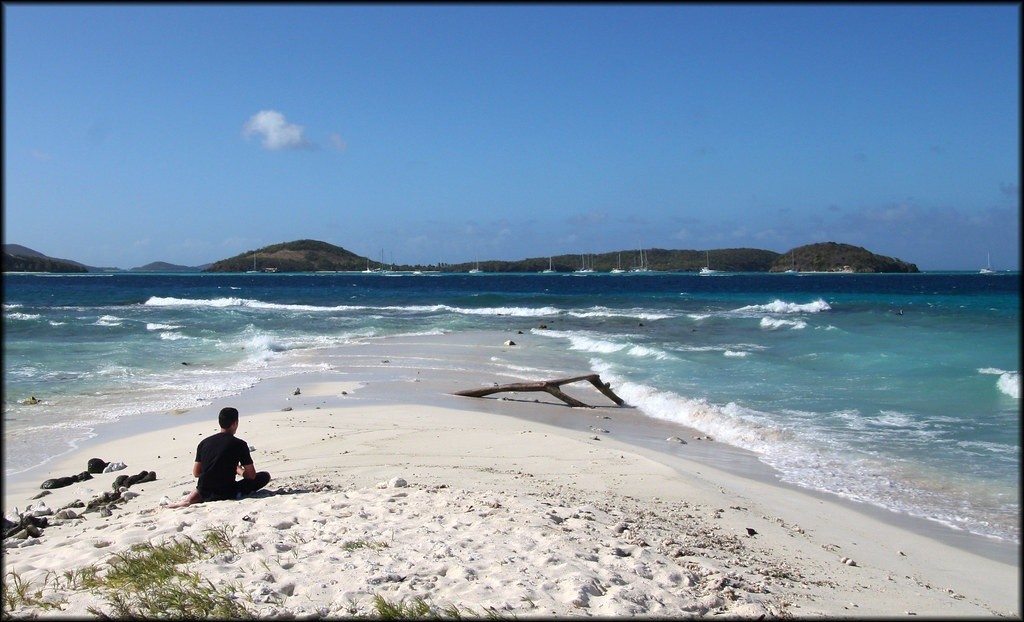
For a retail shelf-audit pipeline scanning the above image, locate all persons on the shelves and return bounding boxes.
[168,407,271,509]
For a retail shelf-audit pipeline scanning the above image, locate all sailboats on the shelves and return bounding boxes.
[541,245,649,274]
[468,255,483,274]
[979,250,995,273]
[359,247,425,277]
[699,251,716,275]
